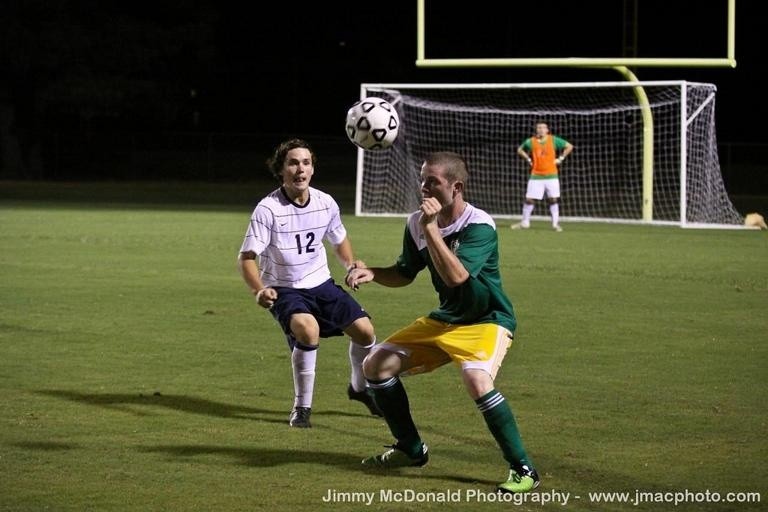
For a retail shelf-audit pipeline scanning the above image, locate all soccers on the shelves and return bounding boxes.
[345,97,400,150]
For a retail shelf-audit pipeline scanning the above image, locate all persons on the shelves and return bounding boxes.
[348,151,539,494]
[510,119,573,233]
[237,138,384,428]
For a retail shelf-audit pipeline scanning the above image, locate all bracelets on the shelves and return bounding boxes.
[527,158,532,162]
[559,155,564,161]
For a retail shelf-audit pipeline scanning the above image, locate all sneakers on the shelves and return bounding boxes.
[346,382,383,417]
[360,442,430,468]
[554,225,561,231]
[498,463,540,492]
[291,407,311,427]
[511,224,527,231]
